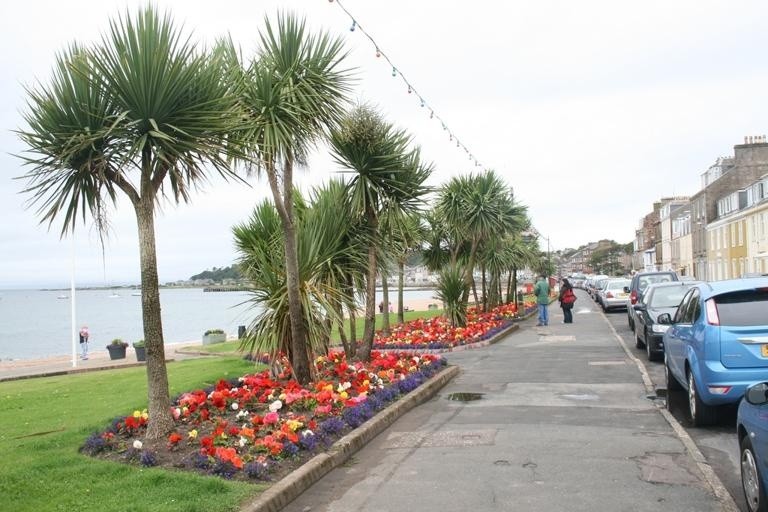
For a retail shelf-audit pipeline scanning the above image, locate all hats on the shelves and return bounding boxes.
[540,274,545,277]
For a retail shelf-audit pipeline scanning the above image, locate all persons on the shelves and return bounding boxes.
[80,327,91,360]
[559,278,574,323]
[534,274,550,325]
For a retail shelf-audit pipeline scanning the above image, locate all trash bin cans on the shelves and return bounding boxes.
[236,325,248,339]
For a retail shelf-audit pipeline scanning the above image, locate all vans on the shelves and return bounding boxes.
[623,272,679,331]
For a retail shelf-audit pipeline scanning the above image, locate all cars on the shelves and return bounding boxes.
[657,271,767,428]
[568,272,632,313]
[736,382,767,512]
[633,281,707,362]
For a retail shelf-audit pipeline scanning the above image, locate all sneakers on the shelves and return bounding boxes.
[536,321,549,326]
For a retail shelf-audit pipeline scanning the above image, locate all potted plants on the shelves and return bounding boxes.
[203,329,226,345]
[108,338,145,361]
